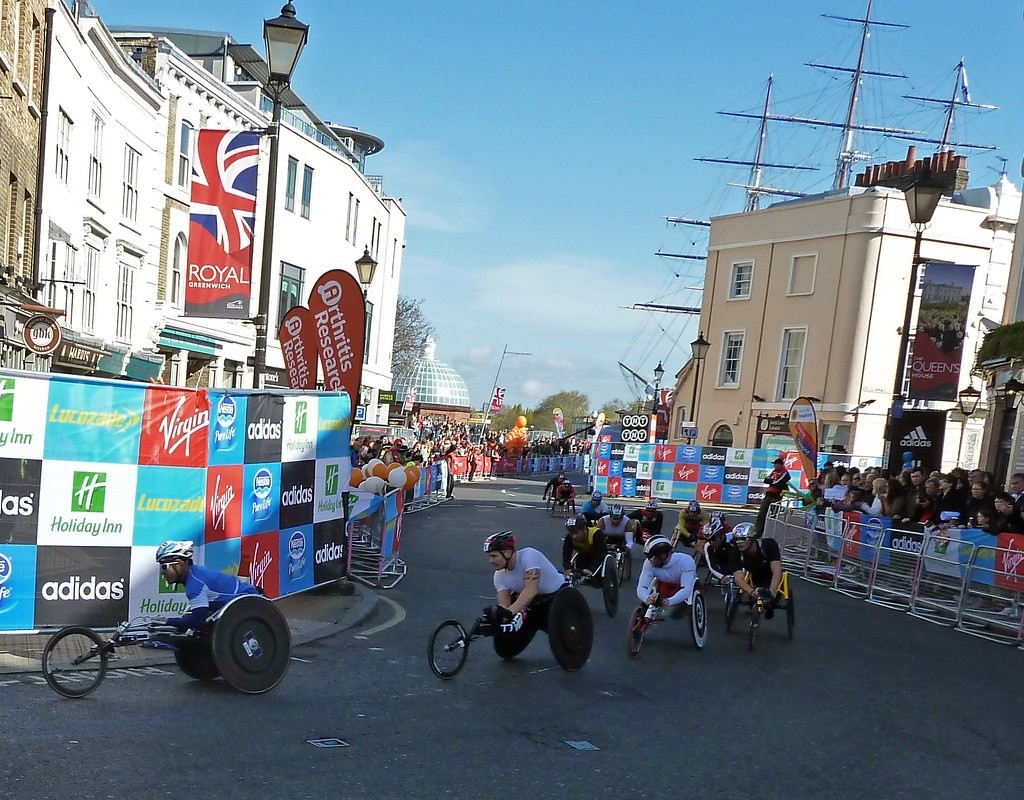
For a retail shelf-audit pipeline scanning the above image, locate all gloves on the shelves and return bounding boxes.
[482,604,513,626]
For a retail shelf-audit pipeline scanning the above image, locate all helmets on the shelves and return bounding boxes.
[558,472,565,479]
[709,511,725,523]
[701,517,724,539]
[562,479,572,489]
[646,500,657,509]
[155,540,194,562]
[610,503,625,518]
[483,530,516,553]
[731,522,758,539]
[643,534,673,557]
[564,515,585,532]
[591,490,603,501]
[688,500,700,512]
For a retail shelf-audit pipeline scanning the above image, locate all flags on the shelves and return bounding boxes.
[961,66,970,104]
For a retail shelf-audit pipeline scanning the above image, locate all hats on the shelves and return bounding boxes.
[771,458,783,464]
[824,461,834,466]
[397,445,410,453]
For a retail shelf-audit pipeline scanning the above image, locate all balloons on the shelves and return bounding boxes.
[349,459,421,494]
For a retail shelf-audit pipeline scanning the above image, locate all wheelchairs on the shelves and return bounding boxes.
[560,550,617,620]
[427,588,592,682]
[724,573,797,653]
[626,578,709,655]
[551,499,577,518]
[43,588,299,694]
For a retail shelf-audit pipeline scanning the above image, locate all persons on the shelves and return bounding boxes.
[678,500,710,549]
[542,471,568,501]
[404,455,423,503]
[556,480,576,506]
[445,445,458,499]
[636,534,698,609]
[596,504,630,551]
[490,445,502,481]
[627,499,664,547]
[698,511,784,620]
[152,540,258,630]
[753,459,791,538]
[468,447,484,482]
[582,491,608,524]
[809,461,1024,617]
[350,413,593,467]
[563,515,609,589]
[481,531,574,630]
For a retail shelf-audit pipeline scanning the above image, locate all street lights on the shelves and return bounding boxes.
[650,360,665,443]
[882,157,944,478]
[241,0,312,392]
[687,329,712,449]
[354,243,380,326]
